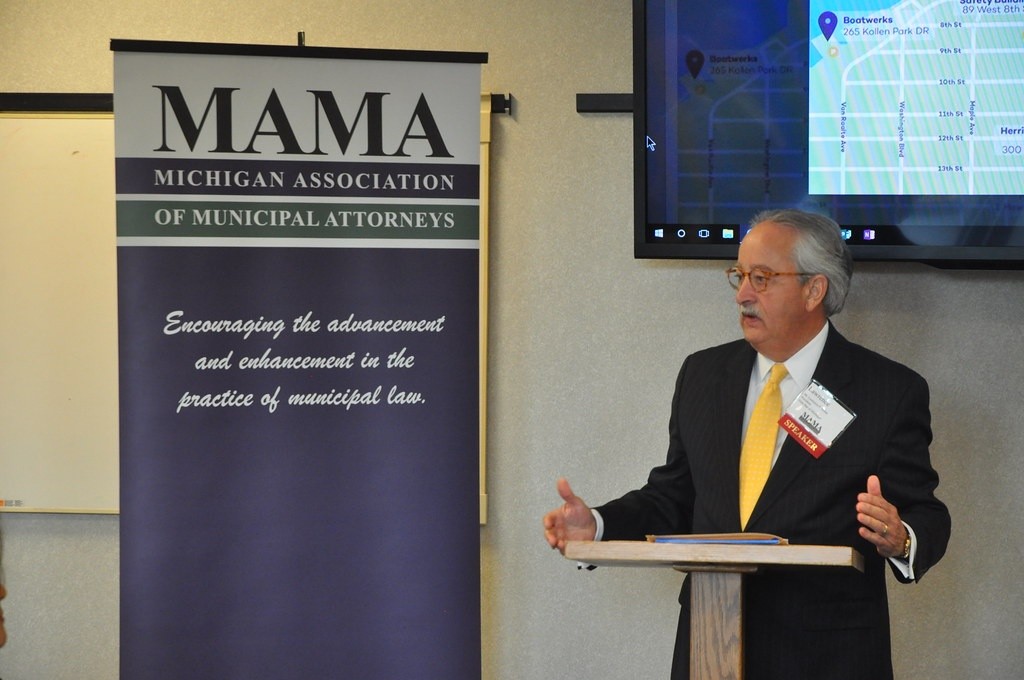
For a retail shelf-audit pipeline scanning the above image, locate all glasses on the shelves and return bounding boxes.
[726,267,829,292]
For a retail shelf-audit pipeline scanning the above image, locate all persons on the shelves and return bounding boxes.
[543,207,951,680]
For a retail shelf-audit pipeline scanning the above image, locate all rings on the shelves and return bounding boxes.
[882,523,888,537]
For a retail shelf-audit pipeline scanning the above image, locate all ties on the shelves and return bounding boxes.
[739,364,788,532]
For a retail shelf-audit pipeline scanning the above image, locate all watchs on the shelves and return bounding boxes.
[892,526,911,560]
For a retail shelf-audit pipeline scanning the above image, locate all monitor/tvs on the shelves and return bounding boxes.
[629,1,1024,271]
